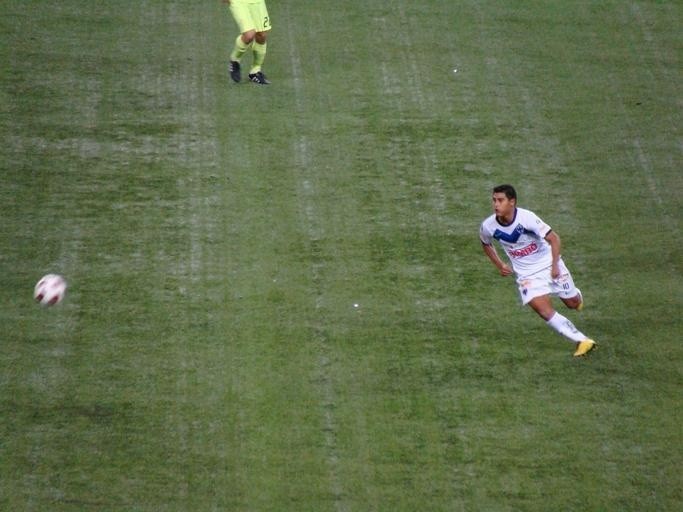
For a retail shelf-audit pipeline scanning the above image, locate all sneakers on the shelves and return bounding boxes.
[571,338,598,358]
[227,58,241,84]
[574,287,585,312]
[246,70,273,86]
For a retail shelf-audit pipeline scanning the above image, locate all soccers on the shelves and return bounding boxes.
[33,274,66,307]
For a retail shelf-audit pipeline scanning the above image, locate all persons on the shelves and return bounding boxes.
[227,0,272,85]
[479,184,598,357]
